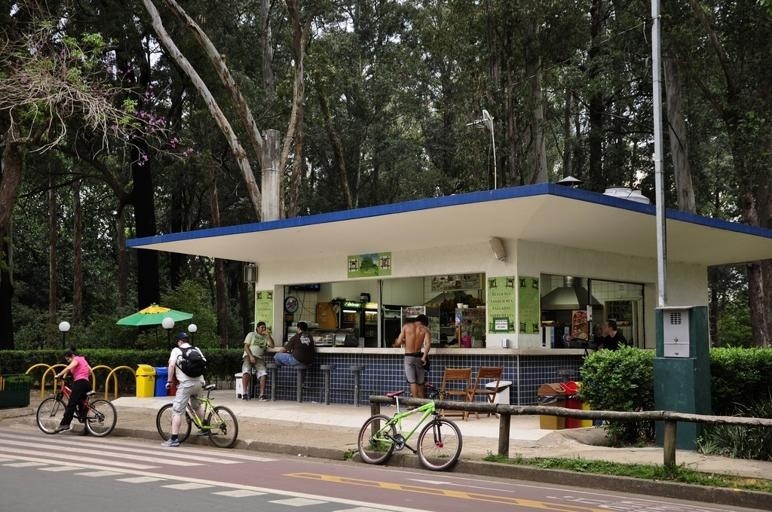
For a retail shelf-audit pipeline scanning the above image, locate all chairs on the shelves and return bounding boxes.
[466,368,503,418]
[439,368,472,421]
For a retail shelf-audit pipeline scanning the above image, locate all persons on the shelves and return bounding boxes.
[160,331,210,448]
[54,351,93,434]
[394,313,432,410]
[594,319,627,352]
[240,321,275,401]
[273,322,313,366]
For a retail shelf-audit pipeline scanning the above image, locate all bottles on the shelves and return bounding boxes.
[55,390,63,402]
[455,312,486,348]
[340,309,379,349]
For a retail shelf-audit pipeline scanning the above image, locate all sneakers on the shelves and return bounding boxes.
[55,424,70,432]
[258,395,268,401]
[161,438,180,447]
[196,430,211,436]
[243,394,248,401]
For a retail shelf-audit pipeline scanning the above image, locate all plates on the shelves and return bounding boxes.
[291,320,319,329]
[311,333,334,346]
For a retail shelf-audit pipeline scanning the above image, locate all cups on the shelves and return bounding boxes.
[462,304,469,309]
[502,338,510,348]
[456,303,463,310]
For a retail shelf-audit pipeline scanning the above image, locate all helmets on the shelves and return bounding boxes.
[175,332,189,341]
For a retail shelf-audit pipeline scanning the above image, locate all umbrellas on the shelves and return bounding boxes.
[115,303,194,345]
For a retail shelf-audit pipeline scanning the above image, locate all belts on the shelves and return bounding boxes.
[405,353,423,357]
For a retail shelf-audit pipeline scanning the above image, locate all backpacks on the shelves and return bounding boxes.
[174,345,205,377]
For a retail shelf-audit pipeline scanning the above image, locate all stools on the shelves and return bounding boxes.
[246,363,364,407]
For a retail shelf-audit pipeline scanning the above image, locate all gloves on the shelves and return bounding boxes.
[165,380,173,390]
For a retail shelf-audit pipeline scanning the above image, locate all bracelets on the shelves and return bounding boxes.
[167,381,173,383]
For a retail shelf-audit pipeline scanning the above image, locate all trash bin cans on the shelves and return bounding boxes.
[135,364,180,397]
[485,380,512,416]
[537,380,610,430]
[234,372,251,400]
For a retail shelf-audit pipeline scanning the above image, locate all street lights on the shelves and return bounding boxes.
[161,317,176,349]
[187,322,198,344]
[479,106,499,192]
[58,320,70,348]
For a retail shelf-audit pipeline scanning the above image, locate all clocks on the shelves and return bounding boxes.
[284,296,299,314]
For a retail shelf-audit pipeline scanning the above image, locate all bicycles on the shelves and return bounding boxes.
[358,381,463,469]
[156,382,239,448]
[36,377,118,437]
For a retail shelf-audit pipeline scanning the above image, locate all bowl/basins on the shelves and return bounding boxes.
[476,305,485,309]
